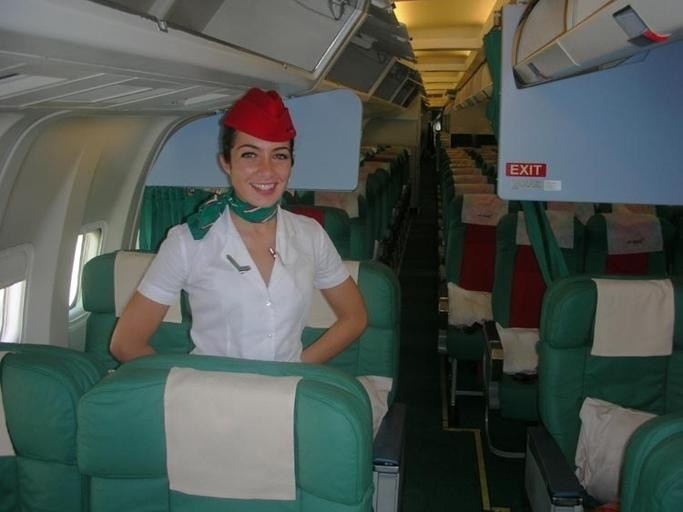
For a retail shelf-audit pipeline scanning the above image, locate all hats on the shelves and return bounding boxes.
[222,88,296,142]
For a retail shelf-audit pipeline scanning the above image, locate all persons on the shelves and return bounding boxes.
[106,86,369,370]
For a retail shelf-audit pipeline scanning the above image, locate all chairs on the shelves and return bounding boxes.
[1,340,103,510]
[81,250,191,355]
[77,351,374,512]
[433,137,673,459]
[201,144,411,262]
[521,271,682,512]
[302,256,411,510]
